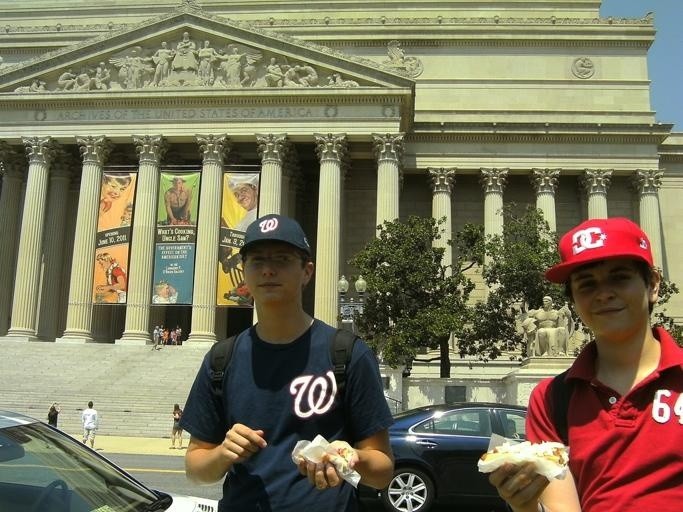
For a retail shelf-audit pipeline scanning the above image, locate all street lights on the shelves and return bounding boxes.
[336,273,367,336]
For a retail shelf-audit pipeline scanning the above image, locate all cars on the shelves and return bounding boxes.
[0,407,219,512]
[353,401,528,512]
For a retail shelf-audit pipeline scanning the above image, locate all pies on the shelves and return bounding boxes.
[299,439,351,476]
[478,440,568,471]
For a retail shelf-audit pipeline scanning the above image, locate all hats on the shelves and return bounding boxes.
[546,217,653,283]
[240,214,310,256]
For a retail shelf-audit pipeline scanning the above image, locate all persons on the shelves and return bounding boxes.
[176,213,398,511]
[487,213,683,512]
[381,40,417,70]
[29,30,320,92]
[81,400,99,452]
[94,167,259,305]
[575,57,594,78]
[167,403,183,450]
[523,295,576,357]
[46,402,61,428]
[150,324,183,351]
